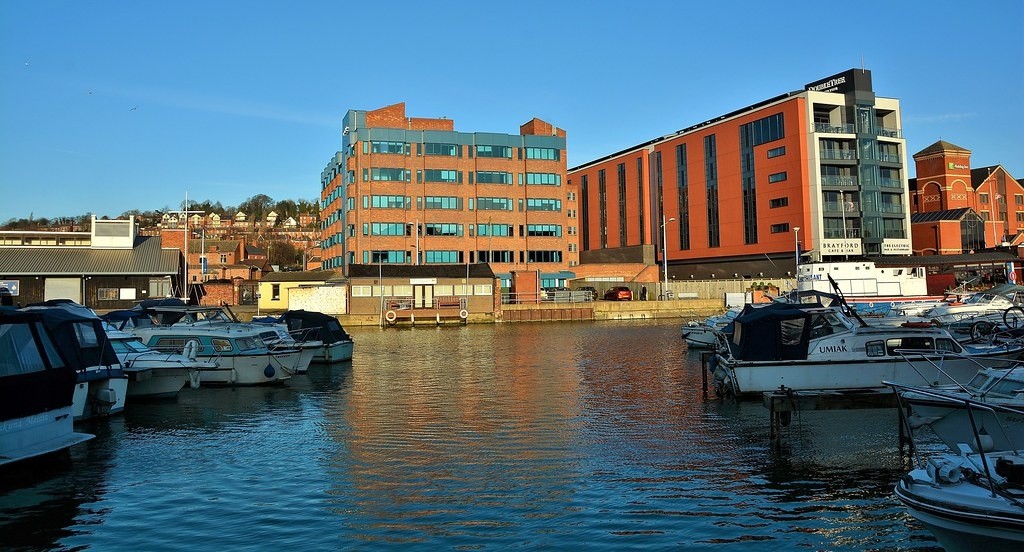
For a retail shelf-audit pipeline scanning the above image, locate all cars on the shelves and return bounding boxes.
[574,285,600,301]
[604,286,632,301]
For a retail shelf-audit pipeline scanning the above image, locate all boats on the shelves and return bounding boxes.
[917,288,1024,371]
[703,288,1024,399]
[881,380,1024,546]
[0,303,96,474]
[881,353,1024,409]
[682,307,740,350]
[35,299,355,420]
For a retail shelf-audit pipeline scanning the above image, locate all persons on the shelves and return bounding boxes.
[594,290,598,301]
[957,278,961,287]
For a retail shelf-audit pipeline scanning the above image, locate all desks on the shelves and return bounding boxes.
[678,292,699,300]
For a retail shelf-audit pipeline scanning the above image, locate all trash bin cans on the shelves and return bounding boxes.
[667,293,674,301]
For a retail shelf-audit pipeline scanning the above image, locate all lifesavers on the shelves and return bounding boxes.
[869,302,874,308]
[1009,271,1017,280]
[901,322,931,327]
[890,301,926,307]
[386,311,397,322]
[182,339,199,360]
[936,300,940,302]
[459,309,468,318]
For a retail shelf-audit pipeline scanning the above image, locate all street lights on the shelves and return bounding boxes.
[407,218,422,268]
[791,224,801,292]
[663,218,676,302]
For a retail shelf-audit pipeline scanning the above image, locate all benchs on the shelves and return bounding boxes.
[437,295,466,310]
[387,296,413,310]
